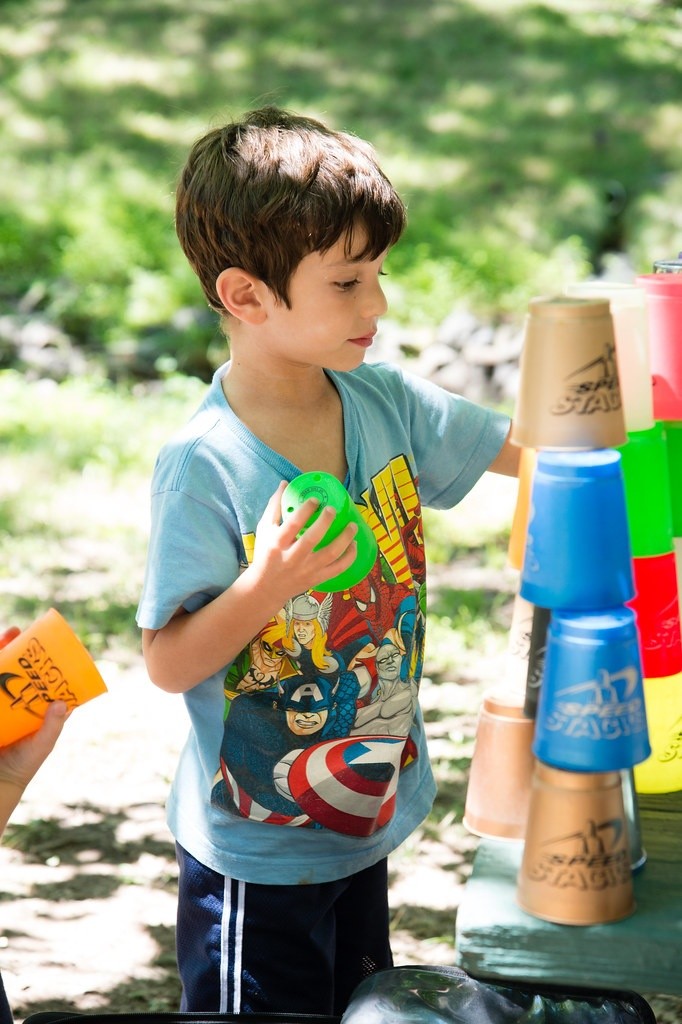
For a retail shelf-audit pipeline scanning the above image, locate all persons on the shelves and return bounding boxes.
[134,107,518,1011]
[0,627,67,1024]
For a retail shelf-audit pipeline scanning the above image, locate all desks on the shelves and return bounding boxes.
[454,794,682,1002]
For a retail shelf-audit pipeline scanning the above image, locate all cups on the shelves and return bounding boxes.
[463,257,679,926]
[281,472,379,591]
[0,607,109,750]
